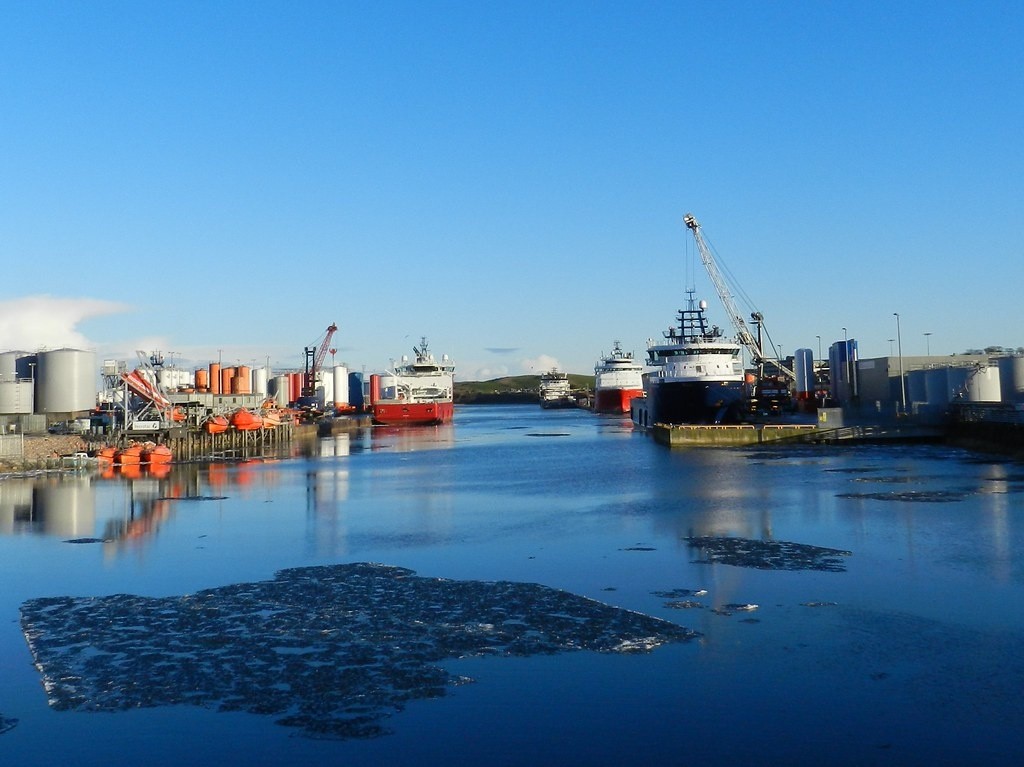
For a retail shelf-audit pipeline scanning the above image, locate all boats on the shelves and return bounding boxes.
[370,335,456,425]
[97,463,173,480]
[591,340,643,414]
[96,441,172,463]
[538,367,571,408]
[203,400,305,433]
[629,212,749,425]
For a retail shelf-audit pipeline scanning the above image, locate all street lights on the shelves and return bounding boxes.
[924,332,932,355]
[894,312,906,411]
[887,339,895,354]
[842,328,849,383]
[816,335,822,388]
[777,345,783,376]
